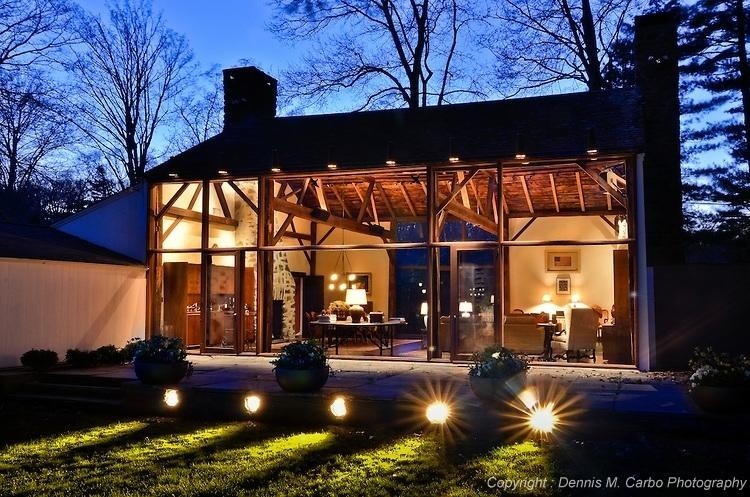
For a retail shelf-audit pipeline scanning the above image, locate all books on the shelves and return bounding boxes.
[318,314,337,324]
[389,317,406,322]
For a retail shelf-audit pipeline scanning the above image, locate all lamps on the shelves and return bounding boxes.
[345,289,368,306]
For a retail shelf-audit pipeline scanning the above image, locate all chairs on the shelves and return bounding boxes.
[439,306,609,364]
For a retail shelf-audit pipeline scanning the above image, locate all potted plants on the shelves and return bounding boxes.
[468,345,529,403]
[128,334,193,398]
[683,345,750,413]
[18,350,60,381]
[270,339,331,395]
[328,300,351,322]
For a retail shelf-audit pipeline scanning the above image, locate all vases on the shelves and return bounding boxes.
[349,305,364,323]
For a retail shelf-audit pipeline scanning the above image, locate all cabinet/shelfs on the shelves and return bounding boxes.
[602,250,631,365]
[163,262,254,351]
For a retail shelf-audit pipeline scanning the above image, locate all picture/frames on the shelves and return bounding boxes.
[345,272,372,296]
[545,246,582,296]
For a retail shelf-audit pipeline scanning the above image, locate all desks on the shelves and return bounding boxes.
[309,315,408,359]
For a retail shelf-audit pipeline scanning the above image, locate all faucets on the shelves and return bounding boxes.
[514,309,524,314]
